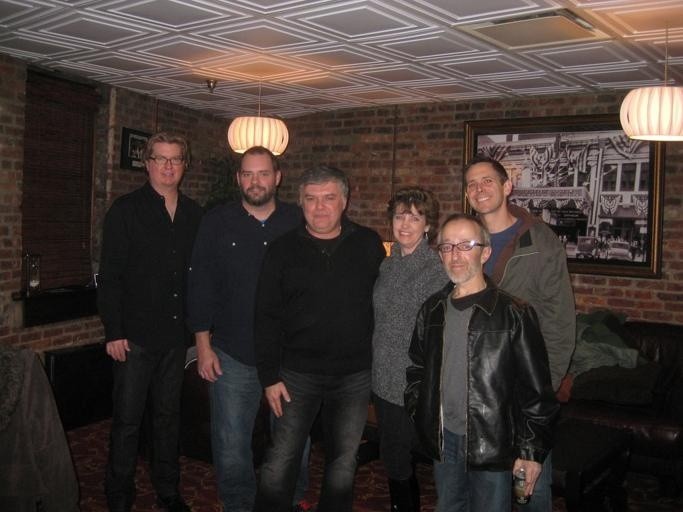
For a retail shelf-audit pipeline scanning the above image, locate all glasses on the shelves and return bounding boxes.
[437,240,484,253]
[149,156,185,166]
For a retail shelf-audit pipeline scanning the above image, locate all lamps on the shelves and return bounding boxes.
[619,19,683,141]
[226,83,290,156]
[25,254,42,297]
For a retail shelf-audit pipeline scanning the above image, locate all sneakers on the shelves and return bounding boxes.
[156,497,192,511]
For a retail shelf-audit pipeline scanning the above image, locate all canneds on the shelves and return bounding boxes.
[513,468,532,504]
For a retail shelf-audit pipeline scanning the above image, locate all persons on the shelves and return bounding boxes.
[95,129,203,512]
[371,187,451,512]
[188,145,314,512]
[402,213,559,511]
[253,164,387,512]
[461,158,578,512]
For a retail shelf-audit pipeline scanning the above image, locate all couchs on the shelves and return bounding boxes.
[555,321,683,499]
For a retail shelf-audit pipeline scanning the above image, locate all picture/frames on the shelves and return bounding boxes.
[463,112,666,280]
